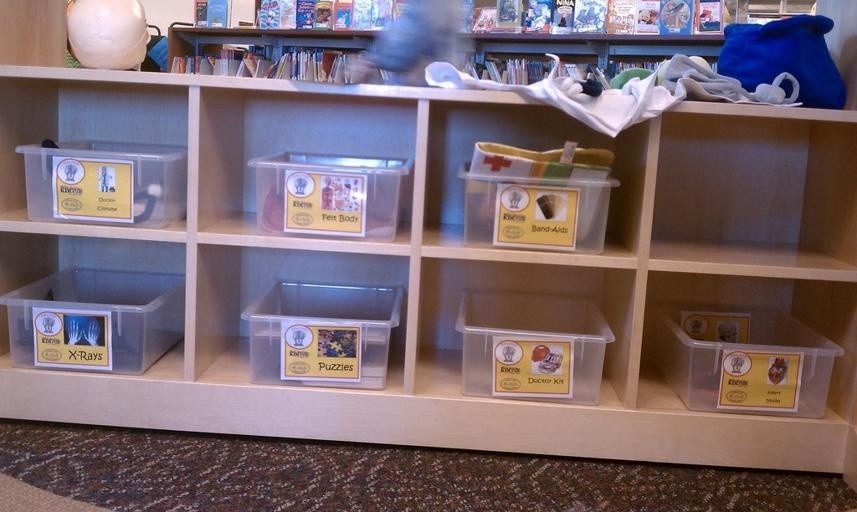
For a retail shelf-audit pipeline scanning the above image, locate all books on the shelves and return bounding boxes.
[169,0,409,85]
[458,0,725,91]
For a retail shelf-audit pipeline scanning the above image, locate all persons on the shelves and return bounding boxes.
[322,176,334,211]
[332,0,463,87]
[98,166,113,192]
[65,319,102,345]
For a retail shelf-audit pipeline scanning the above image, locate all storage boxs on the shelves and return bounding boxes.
[233,278,405,389]
[244,147,413,240]
[659,302,844,418]
[1,267,187,375]
[17,136,188,228]
[455,282,617,405]
[453,169,623,256]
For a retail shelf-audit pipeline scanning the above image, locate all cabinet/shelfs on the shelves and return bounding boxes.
[2,61,855,480]
